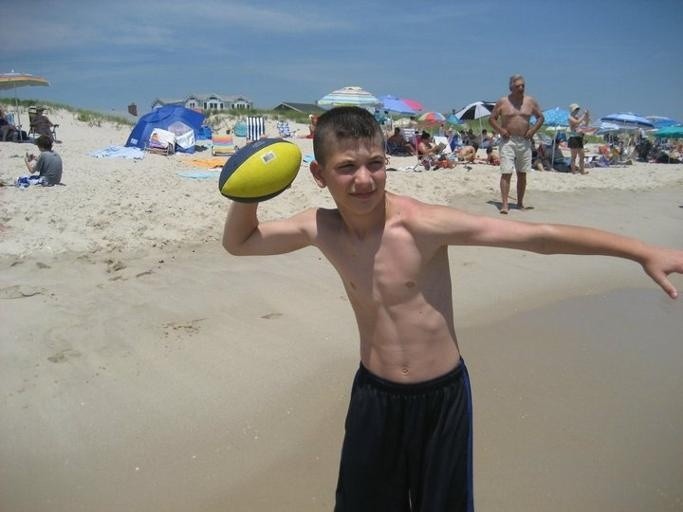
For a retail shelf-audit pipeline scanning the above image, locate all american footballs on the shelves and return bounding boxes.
[218,138,302,204]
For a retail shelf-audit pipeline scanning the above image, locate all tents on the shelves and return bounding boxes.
[122,103,212,154]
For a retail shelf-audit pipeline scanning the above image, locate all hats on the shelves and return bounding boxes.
[569,104,580,112]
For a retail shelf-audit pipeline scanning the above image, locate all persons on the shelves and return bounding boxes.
[565,104,589,174]
[488,74,544,214]
[205,110,683,175]
[221,104,683,512]
[0,111,29,143]
[23,133,63,188]
[31,107,62,144]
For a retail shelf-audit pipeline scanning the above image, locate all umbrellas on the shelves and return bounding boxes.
[0,68,51,140]
